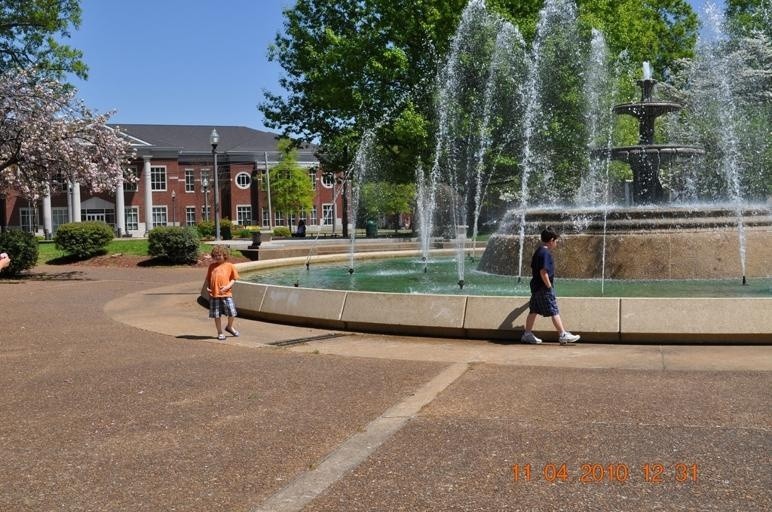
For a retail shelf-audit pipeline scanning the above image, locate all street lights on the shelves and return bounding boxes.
[209,128,221,238]
[171,189,176,227]
[203,178,209,221]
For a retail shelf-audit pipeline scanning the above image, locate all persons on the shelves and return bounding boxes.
[297,220,306,234]
[519,227,582,345]
[0,253,11,271]
[206,244,241,340]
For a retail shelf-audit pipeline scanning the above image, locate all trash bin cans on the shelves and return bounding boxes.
[366,220,377,238]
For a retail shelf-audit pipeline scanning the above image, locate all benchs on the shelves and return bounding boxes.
[304,233,343,238]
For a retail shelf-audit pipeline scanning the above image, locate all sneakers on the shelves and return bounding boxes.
[218,332,225,340]
[521,333,542,344]
[558,332,580,344]
[225,326,240,336]
